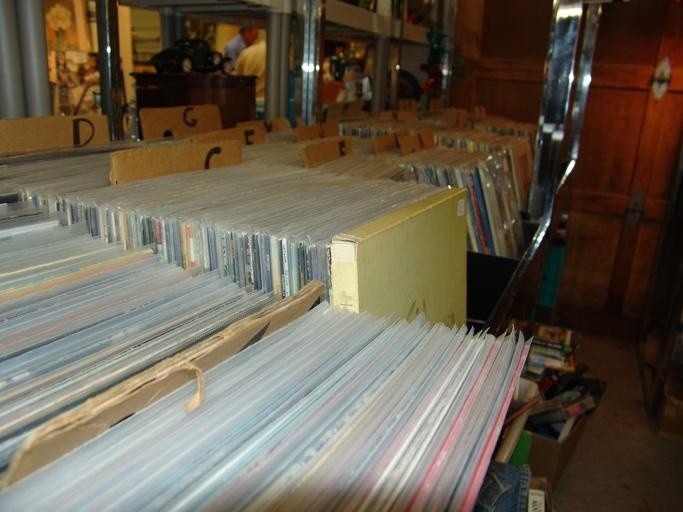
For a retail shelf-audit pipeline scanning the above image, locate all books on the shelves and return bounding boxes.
[502,315,596,442]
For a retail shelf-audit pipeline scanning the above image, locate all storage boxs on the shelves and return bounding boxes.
[494,372,606,499]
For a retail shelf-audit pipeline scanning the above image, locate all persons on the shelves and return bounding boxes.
[234,28,267,100]
[221,25,259,75]
